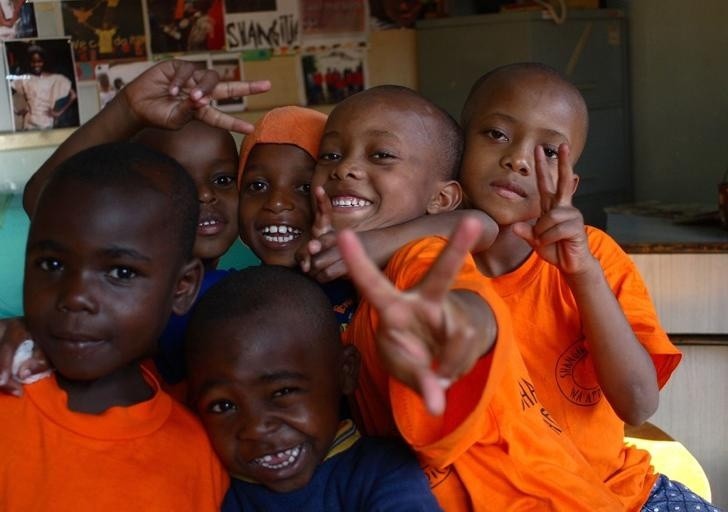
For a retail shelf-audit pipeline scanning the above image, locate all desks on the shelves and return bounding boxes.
[604,209,728,348]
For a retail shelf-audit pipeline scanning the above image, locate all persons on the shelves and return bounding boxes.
[1,140,230,511]
[10,48,77,131]
[86,20,120,58]
[238,106,499,335]
[0,0,27,37]
[310,62,726,512]
[22,59,273,365]
[0,265,443,512]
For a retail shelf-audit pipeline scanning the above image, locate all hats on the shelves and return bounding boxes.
[238,106,327,190]
